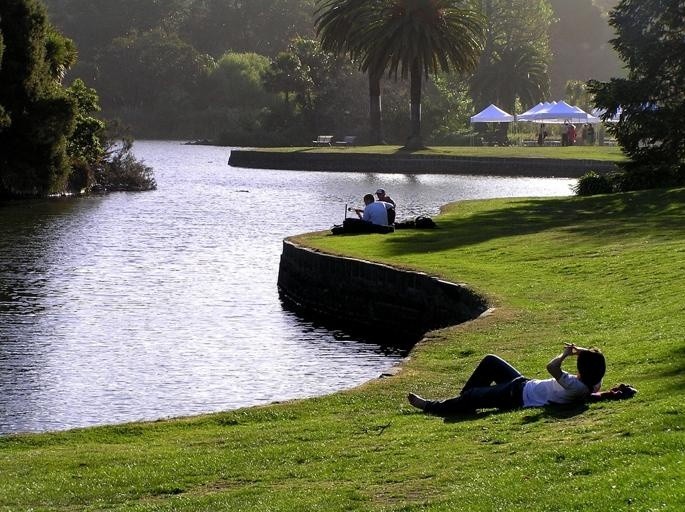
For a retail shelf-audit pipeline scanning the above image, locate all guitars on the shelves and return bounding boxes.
[347,207,395,224]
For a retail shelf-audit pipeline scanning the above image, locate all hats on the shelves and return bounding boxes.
[375,188,384,193]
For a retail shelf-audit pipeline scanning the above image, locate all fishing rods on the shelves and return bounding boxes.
[398,202,419,215]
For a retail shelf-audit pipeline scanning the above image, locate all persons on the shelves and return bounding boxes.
[560,123,596,147]
[375,189,396,225]
[407,342,606,418]
[355,194,394,226]
[539,124,548,147]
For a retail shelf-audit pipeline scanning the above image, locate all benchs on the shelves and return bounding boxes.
[312,136,333,147]
[336,137,353,145]
